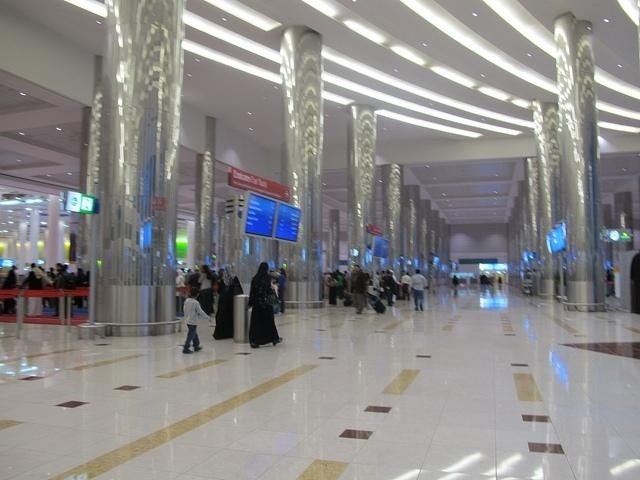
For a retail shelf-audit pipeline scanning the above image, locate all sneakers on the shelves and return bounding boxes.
[182,346,201,353]
[251,343,258,347]
[273,338,282,344]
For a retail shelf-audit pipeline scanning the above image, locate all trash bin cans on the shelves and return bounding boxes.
[233,294,252,343]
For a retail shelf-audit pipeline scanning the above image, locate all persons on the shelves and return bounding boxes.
[175,262,286,353]
[0,262,90,317]
[322,264,536,314]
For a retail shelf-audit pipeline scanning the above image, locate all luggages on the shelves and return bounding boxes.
[366,293,385,313]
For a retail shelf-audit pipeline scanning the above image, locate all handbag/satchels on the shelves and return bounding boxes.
[263,294,275,305]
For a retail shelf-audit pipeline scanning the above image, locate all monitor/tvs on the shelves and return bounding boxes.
[240,190,301,242]
[372,236,391,258]
[59,191,99,215]
[545,220,567,254]
